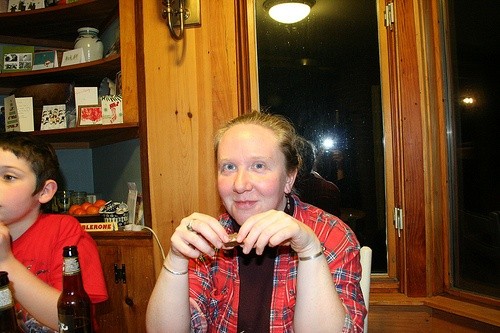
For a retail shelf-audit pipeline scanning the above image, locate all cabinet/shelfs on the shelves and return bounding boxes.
[89,238,157,333]
[0,0,140,143]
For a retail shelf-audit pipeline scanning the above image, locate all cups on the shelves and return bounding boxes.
[42,190,87,213]
[105,200,116,212]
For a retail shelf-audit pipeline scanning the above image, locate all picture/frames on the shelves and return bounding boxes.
[77,105,102,127]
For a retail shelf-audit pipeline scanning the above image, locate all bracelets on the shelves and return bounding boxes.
[162,260,188,276]
[297,242,326,261]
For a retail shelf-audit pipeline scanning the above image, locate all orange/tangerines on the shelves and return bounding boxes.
[68,199,106,215]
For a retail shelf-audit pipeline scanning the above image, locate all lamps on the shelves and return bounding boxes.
[263,0,316,23]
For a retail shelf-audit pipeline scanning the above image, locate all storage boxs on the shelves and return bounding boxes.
[75,87,98,124]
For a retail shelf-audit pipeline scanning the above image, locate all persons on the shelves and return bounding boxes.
[293,137,343,215]
[0,131,108,333]
[318,120,360,202]
[144,112,368,333]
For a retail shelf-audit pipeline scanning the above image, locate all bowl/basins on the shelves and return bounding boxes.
[61,211,101,217]
[8,83,70,107]
[99,212,129,227]
[1,107,43,131]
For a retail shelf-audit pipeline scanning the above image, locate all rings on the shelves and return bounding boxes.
[187,221,194,231]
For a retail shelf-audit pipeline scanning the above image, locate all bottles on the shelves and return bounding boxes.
[57,245,91,333]
[0,271,18,333]
[73,27,104,63]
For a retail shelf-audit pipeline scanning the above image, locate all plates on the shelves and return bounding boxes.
[98,77,116,98]
